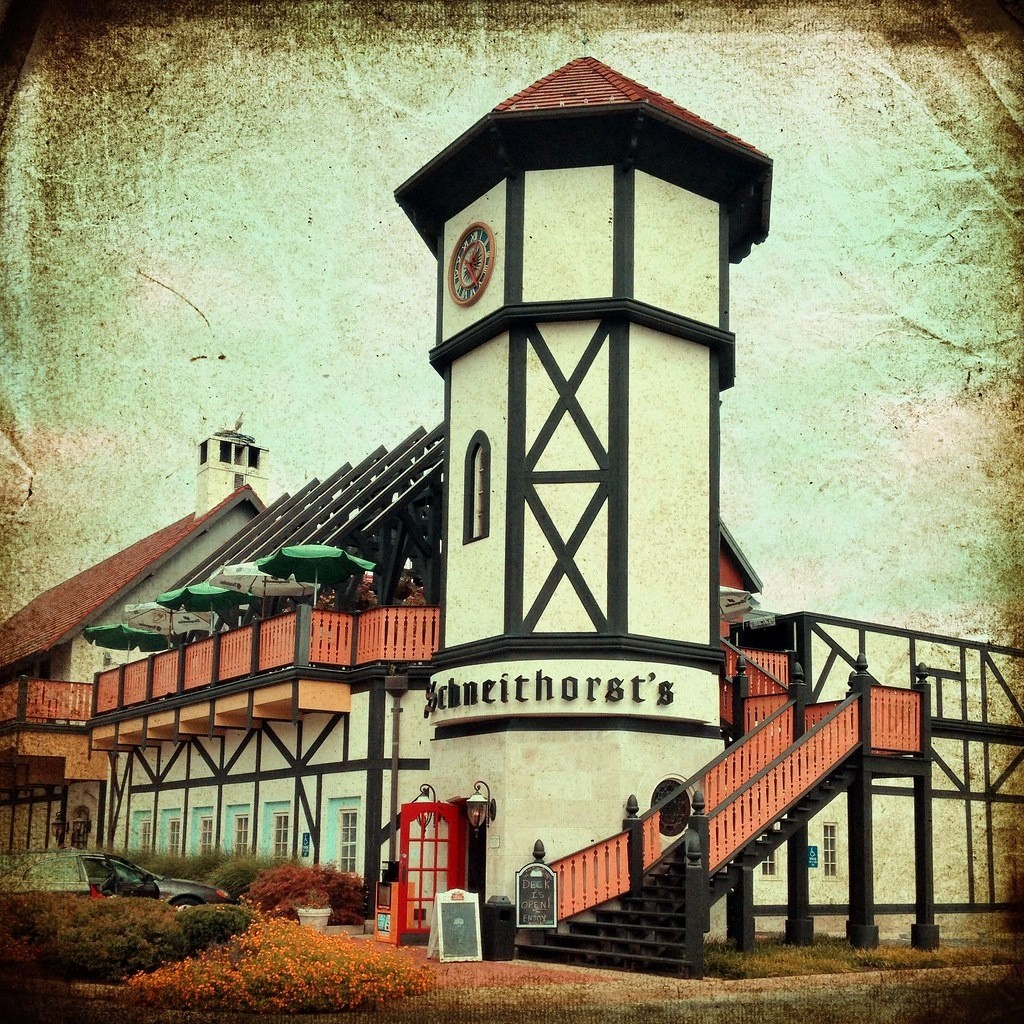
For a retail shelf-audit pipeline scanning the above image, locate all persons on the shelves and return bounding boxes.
[394,569,424,605]
[283,604,292,612]
[317,579,378,610]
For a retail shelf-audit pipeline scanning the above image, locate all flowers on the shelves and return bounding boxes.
[294,888,332,910]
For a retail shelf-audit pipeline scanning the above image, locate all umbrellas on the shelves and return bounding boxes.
[122,602,215,648]
[155,583,262,634]
[83,624,173,661]
[209,562,320,621]
[720,585,761,622]
[253,546,376,608]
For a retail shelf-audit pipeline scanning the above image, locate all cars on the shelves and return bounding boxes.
[0,849,234,910]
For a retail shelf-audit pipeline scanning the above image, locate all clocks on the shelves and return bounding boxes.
[449,222,494,305]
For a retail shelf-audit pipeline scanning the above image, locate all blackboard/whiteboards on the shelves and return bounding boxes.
[515,864,557,928]
[428,890,483,962]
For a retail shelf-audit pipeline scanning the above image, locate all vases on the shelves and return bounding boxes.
[297,908,330,932]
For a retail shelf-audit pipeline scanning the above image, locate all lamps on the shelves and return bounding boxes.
[465,781,499,836]
[51,812,69,843]
[416,785,444,833]
[72,814,90,846]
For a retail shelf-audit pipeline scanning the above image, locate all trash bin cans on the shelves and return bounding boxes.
[485,896,516,961]
